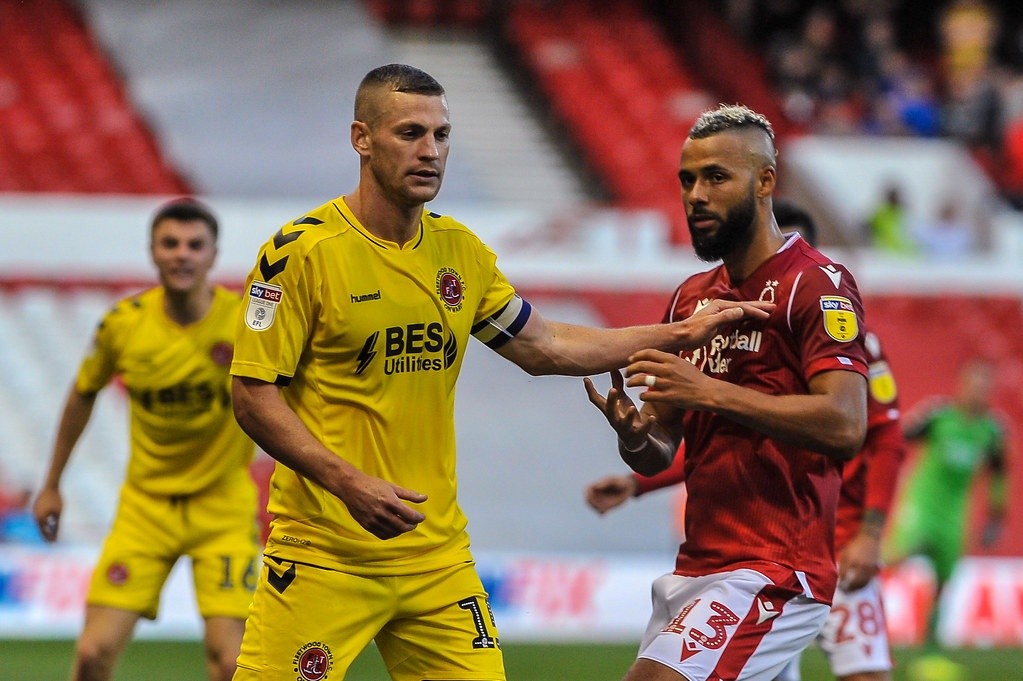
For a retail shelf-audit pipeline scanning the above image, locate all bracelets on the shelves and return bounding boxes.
[616,434,649,452]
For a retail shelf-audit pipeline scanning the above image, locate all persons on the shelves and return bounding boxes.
[876,348,1008,679]
[583,105,869,681]
[229,63,778,681]
[30,198,261,680]
[590,199,900,681]
[761,0,1023,209]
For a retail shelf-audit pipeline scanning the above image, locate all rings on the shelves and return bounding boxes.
[645,375,657,386]
